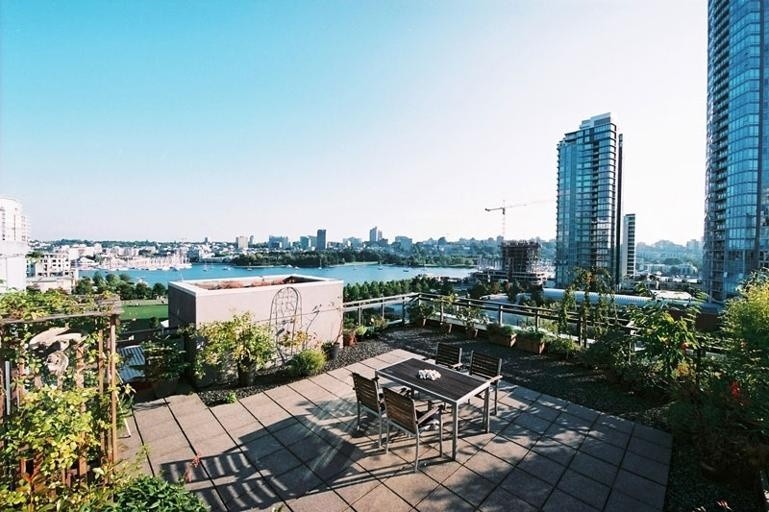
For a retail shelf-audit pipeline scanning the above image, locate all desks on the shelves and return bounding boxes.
[370,356,496,465]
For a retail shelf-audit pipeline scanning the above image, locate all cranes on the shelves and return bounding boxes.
[484,198,541,240]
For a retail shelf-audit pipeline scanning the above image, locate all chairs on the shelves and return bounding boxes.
[466,350,509,418]
[424,342,464,370]
[349,369,398,448]
[380,384,452,473]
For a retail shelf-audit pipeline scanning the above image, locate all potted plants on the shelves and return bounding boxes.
[320,336,343,361]
[187,305,281,391]
[435,291,462,334]
[483,321,546,353]
[457,304,486,340]
[400,300,435,329]
[341,320,364,347]
